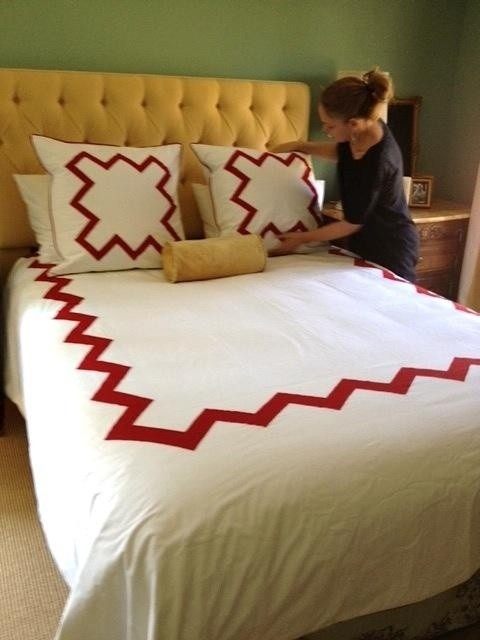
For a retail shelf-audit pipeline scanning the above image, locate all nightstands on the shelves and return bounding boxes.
[322,197,471,302]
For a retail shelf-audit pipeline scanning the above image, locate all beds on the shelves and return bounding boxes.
[0,67,480,639]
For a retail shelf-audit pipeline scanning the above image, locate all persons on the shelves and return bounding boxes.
[268,69,419,282]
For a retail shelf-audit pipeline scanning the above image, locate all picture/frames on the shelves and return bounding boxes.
[406,176,433,212]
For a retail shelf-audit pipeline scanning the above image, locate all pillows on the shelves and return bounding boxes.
[11,135,325,284]
